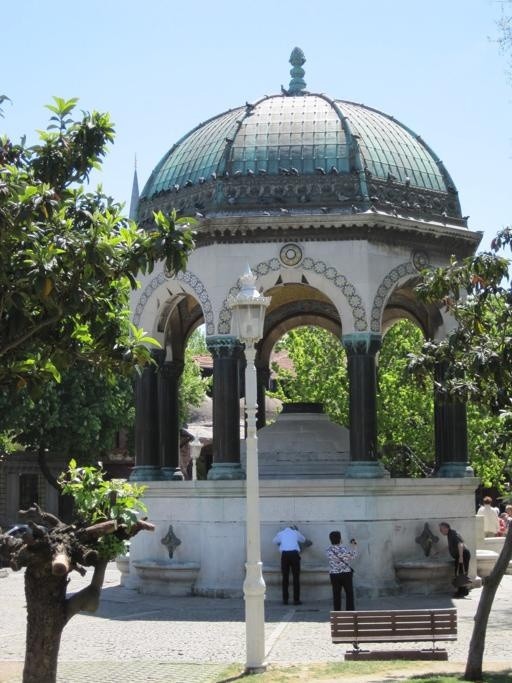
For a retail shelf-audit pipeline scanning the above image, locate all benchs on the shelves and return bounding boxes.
[329,609,459,661]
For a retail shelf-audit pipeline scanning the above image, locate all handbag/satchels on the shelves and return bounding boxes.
[451,572,473,588]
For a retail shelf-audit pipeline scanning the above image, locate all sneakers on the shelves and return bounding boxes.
[452,589,468,598]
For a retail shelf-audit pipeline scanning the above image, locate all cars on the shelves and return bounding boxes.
[1,520,49,537]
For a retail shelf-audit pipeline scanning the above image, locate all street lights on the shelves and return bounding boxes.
[183,428,206,483]
[226,265,274,674]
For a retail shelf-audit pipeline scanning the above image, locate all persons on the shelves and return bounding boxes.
[272,525,313,605]
[504,503,511,525]
[493,506,506,537]
[439,521,470,596]
[326,530,361,611]
[477,495,499,537]
[500,513,512,527]
